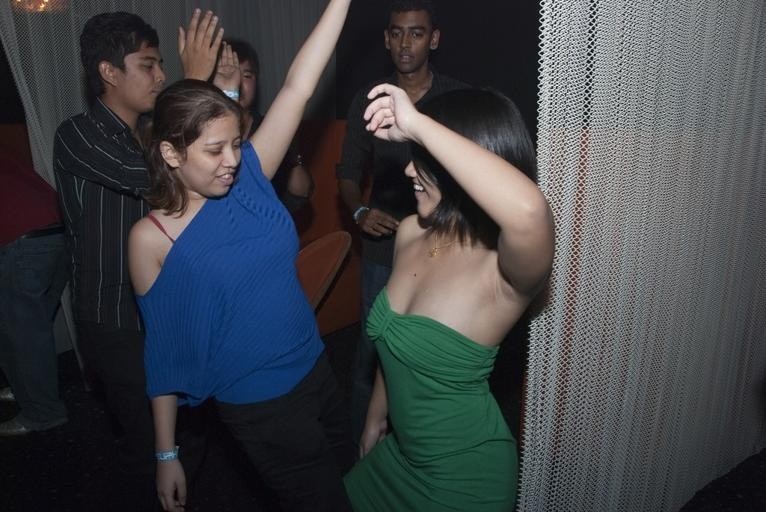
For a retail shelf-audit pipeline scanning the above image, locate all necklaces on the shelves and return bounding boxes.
[426,231,466,259]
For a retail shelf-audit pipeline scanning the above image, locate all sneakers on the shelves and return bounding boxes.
[0,386,18,402]
[0,407,69,439]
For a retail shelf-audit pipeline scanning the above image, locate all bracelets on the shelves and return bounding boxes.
[352,203,370,226]
[150,445,180,465]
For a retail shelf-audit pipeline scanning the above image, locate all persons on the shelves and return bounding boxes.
[336,2,474,318]
[211,37,316,212]
[123,0,353,511]
[1,120,73,436]
[341,79,556,512]
[53,7,242,511]
